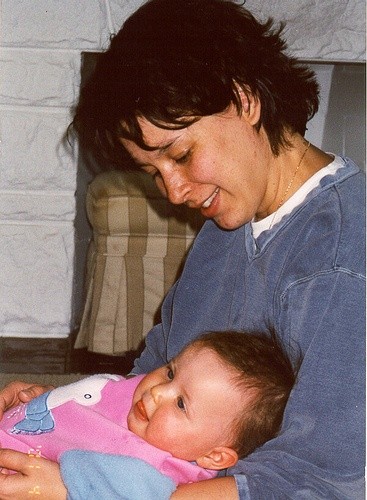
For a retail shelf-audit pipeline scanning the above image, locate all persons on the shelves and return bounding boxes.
[0,320,303,500]
[0,0,365,500]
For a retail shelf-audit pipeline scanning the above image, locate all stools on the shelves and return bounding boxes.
[74,164,206,358]
